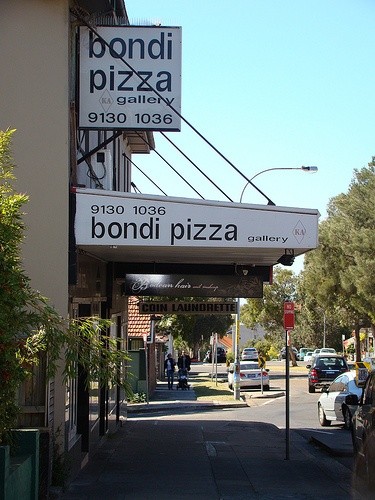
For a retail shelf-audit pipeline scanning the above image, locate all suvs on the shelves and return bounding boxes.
[208,347,227,363]
[306,355,352,393]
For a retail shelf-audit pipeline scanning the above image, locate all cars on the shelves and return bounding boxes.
[241,347,260,362]
[281,347,338,367]
[317,370,363,432]
[344,369,375,492]
[226,360,269,391]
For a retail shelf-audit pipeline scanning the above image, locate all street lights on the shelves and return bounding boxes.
[234,165,319,400]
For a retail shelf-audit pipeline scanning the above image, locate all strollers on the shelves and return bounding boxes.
[177,368,191,391]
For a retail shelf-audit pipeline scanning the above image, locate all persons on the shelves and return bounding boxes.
[177,351,192,387]
[165,353,175,391]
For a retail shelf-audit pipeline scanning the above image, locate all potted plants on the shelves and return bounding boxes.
[0,123,141,500]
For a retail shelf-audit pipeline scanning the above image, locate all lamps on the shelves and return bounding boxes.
[277,248,296,266]
[239,166,318,203]
[240,263,250,277]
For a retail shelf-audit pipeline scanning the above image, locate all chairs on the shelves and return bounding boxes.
[320,360,327,370]
[335,360,343,367]
